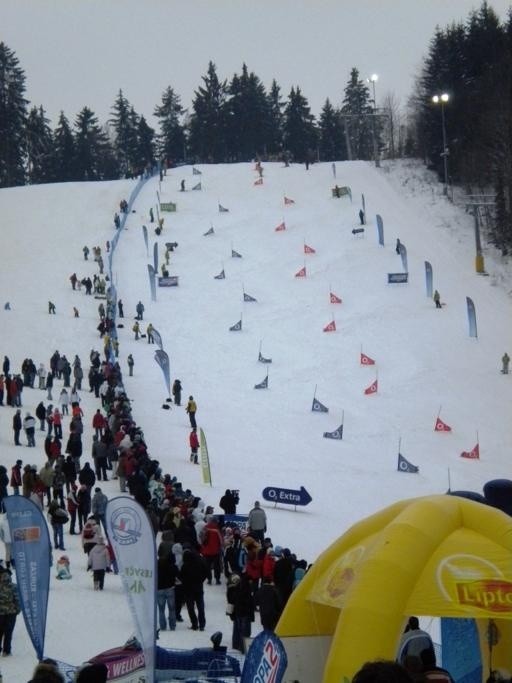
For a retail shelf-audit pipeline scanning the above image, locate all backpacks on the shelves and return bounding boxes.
[84,522,96,539]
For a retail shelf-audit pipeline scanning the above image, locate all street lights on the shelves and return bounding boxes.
[432,92,451,193]
[366,73,384,164]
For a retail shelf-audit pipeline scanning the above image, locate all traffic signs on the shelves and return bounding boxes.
[259,484,313,506]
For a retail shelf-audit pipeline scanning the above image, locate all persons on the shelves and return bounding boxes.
[125,152,313,192]
[433,289,442,308]
[359,209,364,225]
[395,238,400,255]
[335,185,340,198]
[502,353,510,374]
[351,616,457,683]
[5,199,178,349]
[1,343,313,682]
